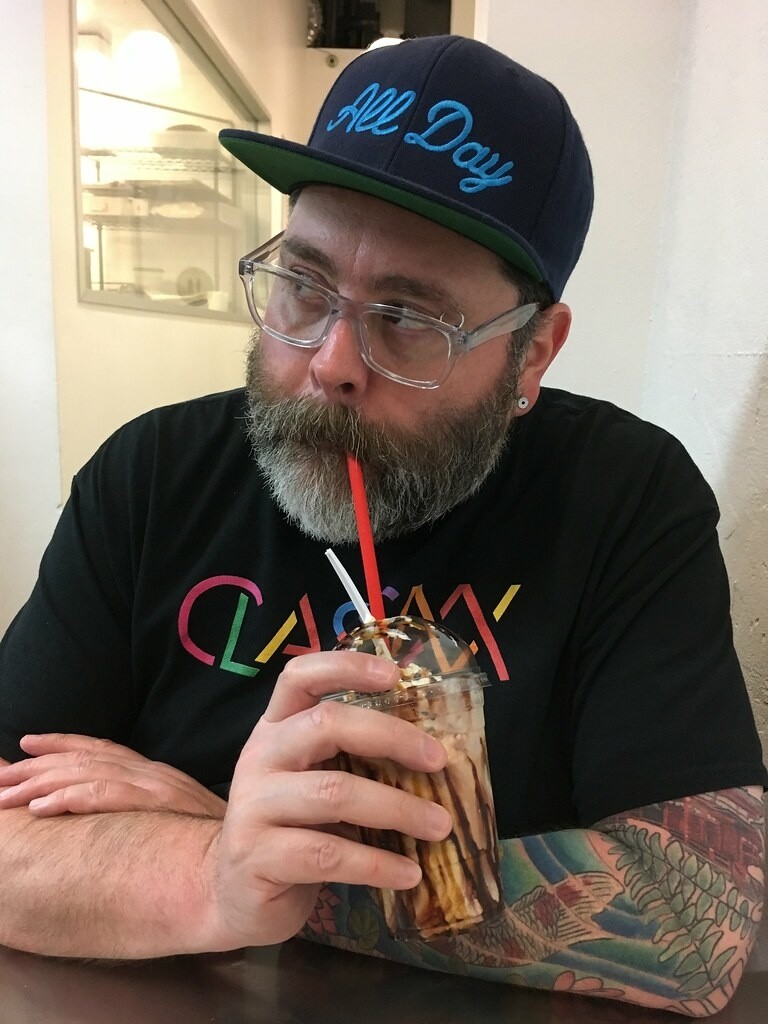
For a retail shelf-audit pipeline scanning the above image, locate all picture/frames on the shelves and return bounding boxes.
[64,0,488,337]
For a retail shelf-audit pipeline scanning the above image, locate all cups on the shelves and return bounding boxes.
[319,616,500,946]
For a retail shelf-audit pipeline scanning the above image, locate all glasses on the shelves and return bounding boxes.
[239,228,541,390]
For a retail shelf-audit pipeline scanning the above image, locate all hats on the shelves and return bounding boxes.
[219,34,594,305]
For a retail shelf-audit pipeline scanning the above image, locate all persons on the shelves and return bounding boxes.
[0,35,766,1017]
